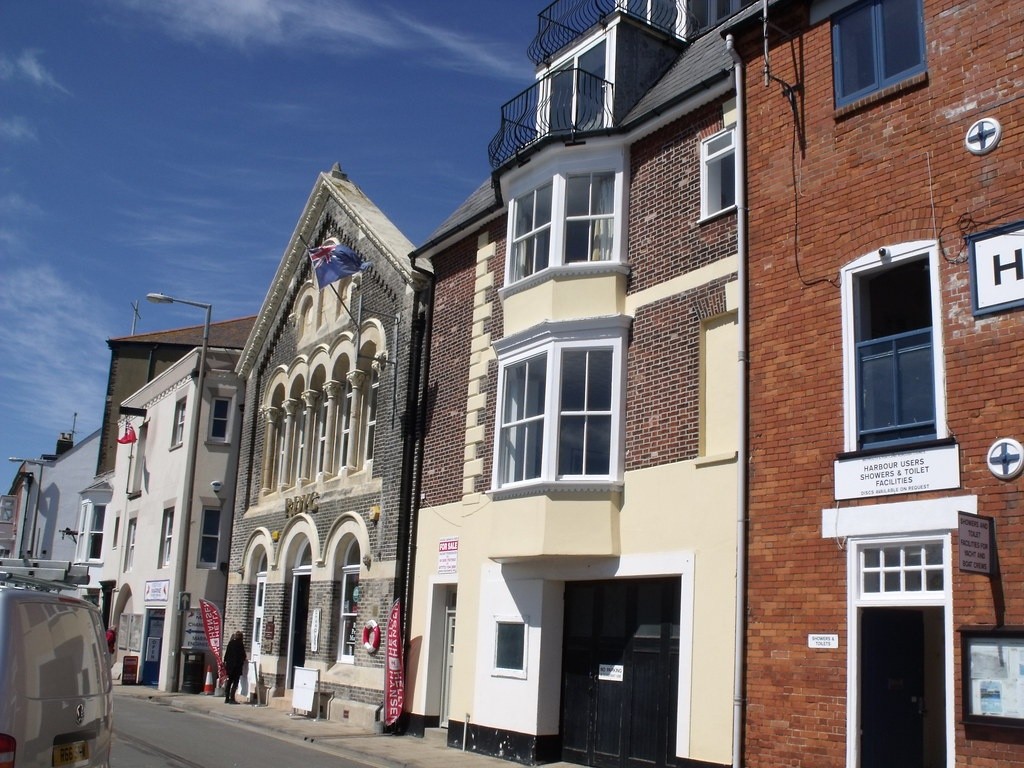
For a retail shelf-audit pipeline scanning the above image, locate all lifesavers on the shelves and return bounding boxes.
[362,619,381,654]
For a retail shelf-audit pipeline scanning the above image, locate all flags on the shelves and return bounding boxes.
[116,422,136,444]
[307,244,372,291]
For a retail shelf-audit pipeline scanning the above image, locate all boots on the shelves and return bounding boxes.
[224,689,239,704]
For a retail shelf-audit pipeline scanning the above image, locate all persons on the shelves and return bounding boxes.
[224,631,247,704]
[107,625,116,656]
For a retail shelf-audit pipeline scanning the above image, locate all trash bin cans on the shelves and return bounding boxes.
[182,650,205,694]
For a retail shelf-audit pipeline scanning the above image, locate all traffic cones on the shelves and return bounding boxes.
[204,665,215,695]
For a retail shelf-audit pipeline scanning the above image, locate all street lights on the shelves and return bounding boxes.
[8,456,45,557]
[145,293,213,693]
